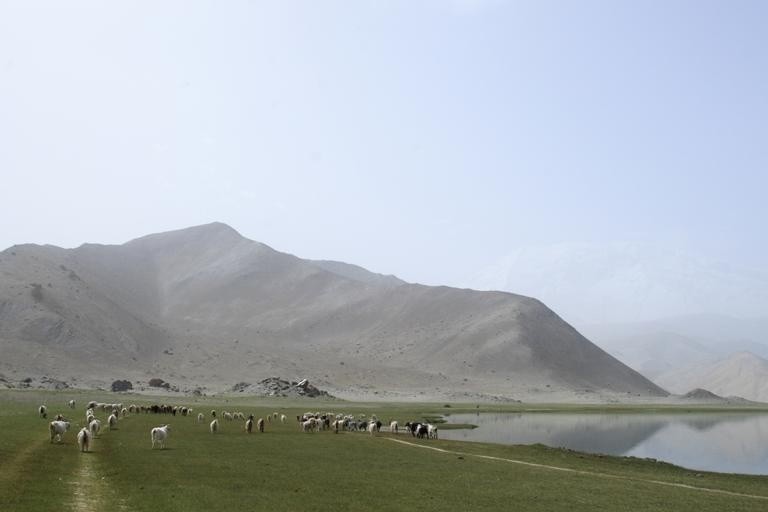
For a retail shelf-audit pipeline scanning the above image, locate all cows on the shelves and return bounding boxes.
[198,413,204,423]
[39,405,47,418]
[77,415,101,453]
[68,400,76,408]
[49,414,70,444]
[297,412,438,441]
[210,409,287,433]
[150,424,172,449]
[108,414,117,432]
[84,401,194,418]
